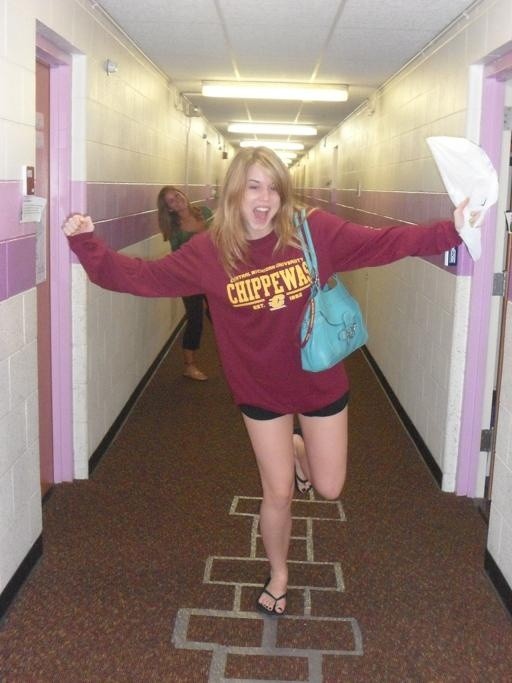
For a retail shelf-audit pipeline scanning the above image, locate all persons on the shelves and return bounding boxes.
[155,186,216,384]
[61,143,487,618]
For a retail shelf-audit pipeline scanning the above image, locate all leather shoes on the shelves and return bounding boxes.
[183,366,209,381]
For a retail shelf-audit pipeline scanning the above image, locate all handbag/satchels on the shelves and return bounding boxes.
[293,206,370,373]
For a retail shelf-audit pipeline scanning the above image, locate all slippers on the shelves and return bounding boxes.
[257,572,286,616]
[292,428,314,493]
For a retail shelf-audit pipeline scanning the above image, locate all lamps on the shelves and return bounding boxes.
[199,80,349,102]
[227,121,317,168]
